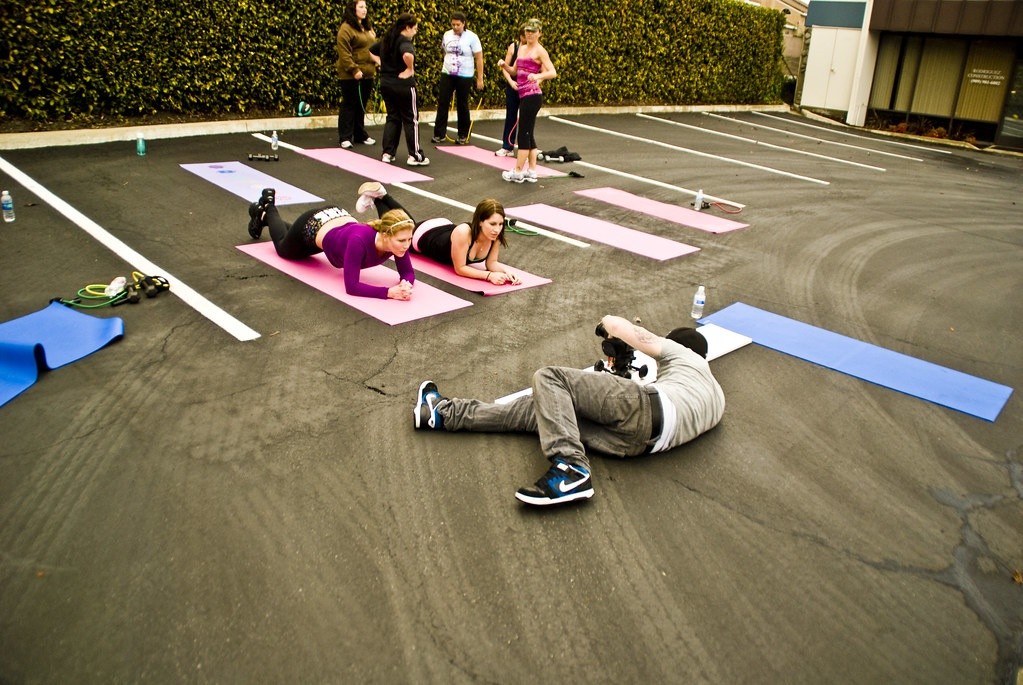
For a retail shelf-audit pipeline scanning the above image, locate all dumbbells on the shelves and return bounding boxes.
[249,154,269,161]
[258,153,279,161]
[537,153,564,163]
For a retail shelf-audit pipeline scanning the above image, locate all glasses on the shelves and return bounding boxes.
[357,6,368,10]
[413,27,418,31]
[451,23,463,27]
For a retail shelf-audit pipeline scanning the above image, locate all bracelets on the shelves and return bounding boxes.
[485,271,493,281]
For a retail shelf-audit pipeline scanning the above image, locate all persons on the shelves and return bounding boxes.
[243,187,415,301]
[410,314,727,509]
[369,13,431,166]
[495,18,557,184]
[355,181,523,288]
[431,12,484,145]
[336,0,380,150]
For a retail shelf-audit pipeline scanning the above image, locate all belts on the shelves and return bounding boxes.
[640,386,660,456]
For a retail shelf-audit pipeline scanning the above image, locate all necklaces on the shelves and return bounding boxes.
[478,237,489,252]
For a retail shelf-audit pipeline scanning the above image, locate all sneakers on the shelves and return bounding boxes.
[382,153,396,163]
[407,149,429,165]
[413,380,450,431]
[364,137,376,145]
[248,188,276,240]
[356,181,386,213]
[515,460,594,505]
[455,137,470,145]
[502,169,524,184]
[431,136,446,143]
[342,141,354,149]
[495,148,514,157]
[523,168,538,183]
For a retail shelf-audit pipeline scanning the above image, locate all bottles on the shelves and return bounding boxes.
[136,133,147,155]
[0,190,15,223]
[694,189,704,211]
[104,276,126,296]
[690,285,706,319]
[271,131,279,151]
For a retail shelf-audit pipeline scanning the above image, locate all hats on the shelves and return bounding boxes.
[524,18,543,31]
